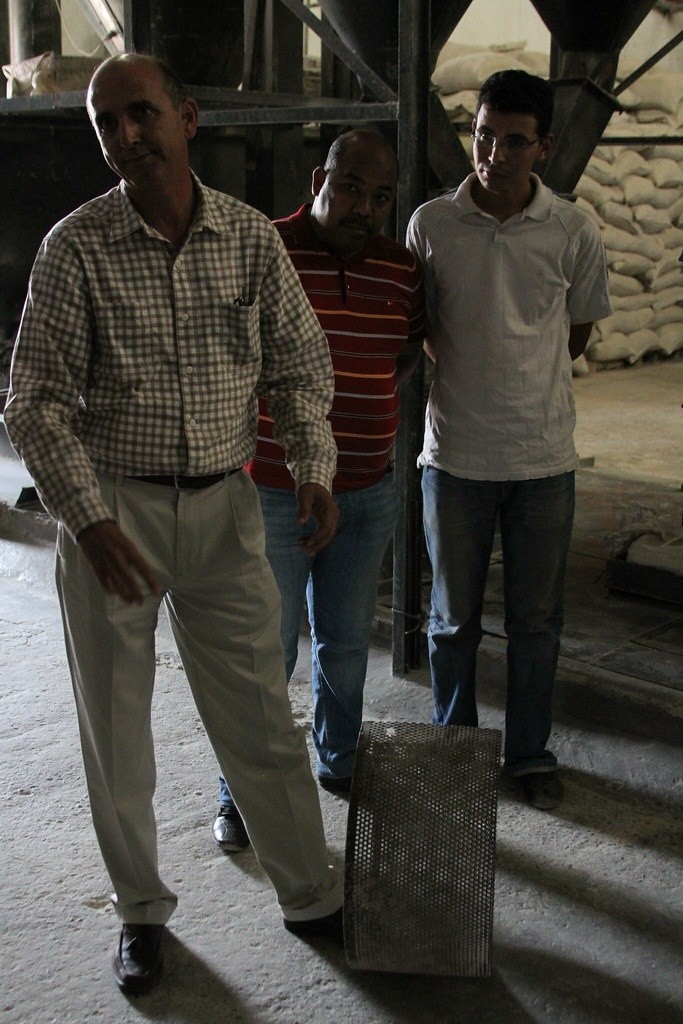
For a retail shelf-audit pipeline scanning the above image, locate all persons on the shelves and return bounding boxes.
[404,67,616,810]
[210,126,427,856]
[1,52,346,986]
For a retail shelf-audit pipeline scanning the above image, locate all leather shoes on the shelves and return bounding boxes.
[525,770,564,810]
[110,923,165,994]
[283,904,344,938]
[212,805,249,854]
[318,773,353,792]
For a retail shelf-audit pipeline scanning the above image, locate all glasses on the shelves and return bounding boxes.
[471,127,545,154]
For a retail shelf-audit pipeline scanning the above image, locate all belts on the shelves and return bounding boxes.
[126,465,245,491]
